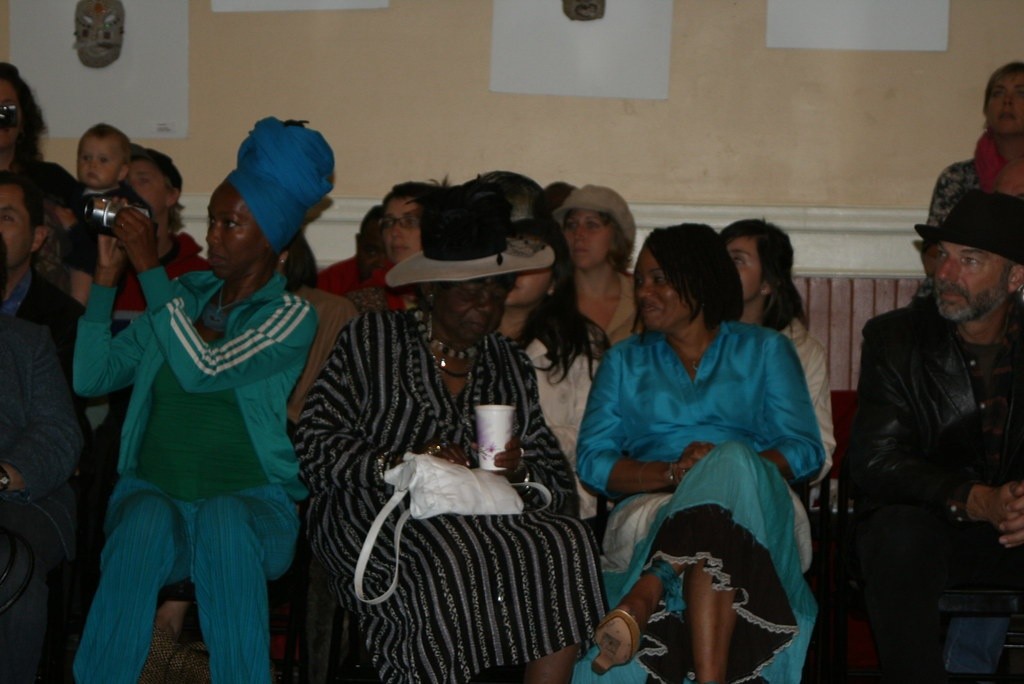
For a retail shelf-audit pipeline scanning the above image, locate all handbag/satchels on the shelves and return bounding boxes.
[355,451,550,606]
[133,622,276,684]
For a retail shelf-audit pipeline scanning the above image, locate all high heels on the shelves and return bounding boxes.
[592,608,639,675]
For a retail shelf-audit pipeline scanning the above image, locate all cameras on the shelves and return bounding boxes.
[84,198,150,229]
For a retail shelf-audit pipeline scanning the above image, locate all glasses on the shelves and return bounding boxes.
[378,214,422,229]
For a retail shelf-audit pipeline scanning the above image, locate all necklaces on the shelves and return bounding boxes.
[433,356,469,377]
[693,360,698,371]
[215,289,241,319]
[414,310,480,358]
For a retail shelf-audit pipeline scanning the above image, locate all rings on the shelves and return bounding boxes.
[427,441,447,456]
[520,448,524,457]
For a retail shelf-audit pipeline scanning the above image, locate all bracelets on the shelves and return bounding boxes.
[0,466,10,490]
[121,221,127,228]
[379,451,389,478]
[670,463,676,485]
[517,466,532,493]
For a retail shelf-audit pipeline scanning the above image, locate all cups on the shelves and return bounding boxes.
[474,404,518,470]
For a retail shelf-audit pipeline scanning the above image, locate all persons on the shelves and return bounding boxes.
[316,182,431,312]
[576,223,827,684]
[501,219,610,550]
[851,188,1024,684]
[921,62,1024,276]
[721,218,835,484]
[294,171,608,684]
[0,63,213,684]
[287,231,355,437]
[552,185,641,345]
[73,118,335,684]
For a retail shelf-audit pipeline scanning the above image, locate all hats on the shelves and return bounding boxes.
[385,184,556,288]
[914,189,1024,263]
[551,185,636,244]
